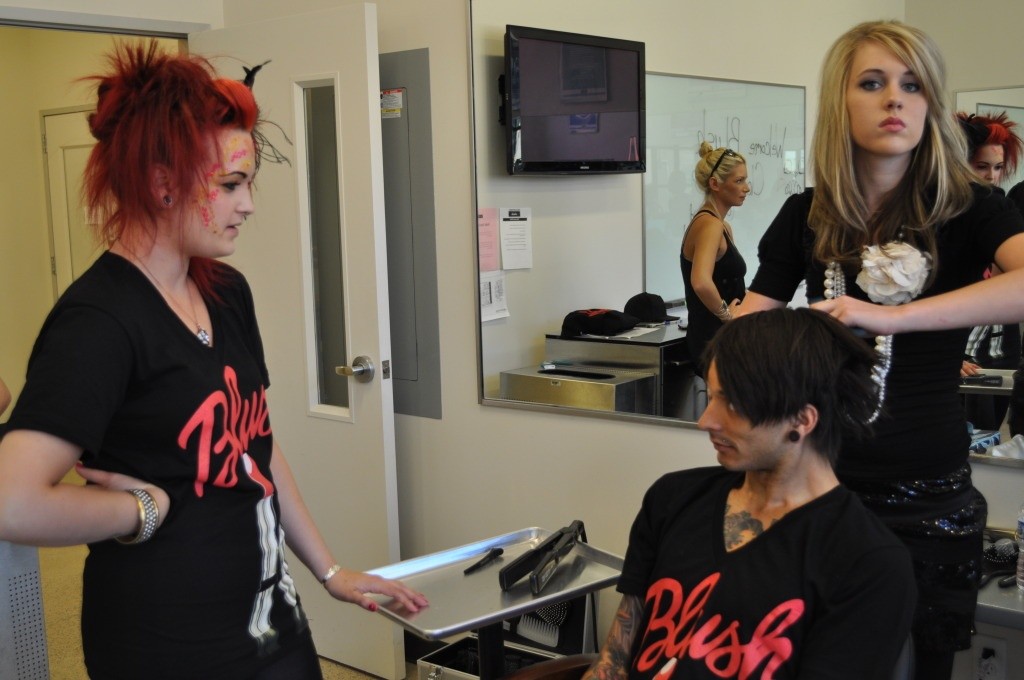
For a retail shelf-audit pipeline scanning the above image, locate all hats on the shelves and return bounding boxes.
[624,292,680,323]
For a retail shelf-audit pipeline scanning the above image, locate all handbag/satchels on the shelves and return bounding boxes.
[560,309,635,338]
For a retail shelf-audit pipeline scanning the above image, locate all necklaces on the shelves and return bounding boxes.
[117,236,209,345]
[824,223,906,425]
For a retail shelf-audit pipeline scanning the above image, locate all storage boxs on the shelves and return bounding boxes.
[415,590,600,680]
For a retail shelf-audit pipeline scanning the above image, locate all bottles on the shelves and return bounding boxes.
[1016,505,1024,591]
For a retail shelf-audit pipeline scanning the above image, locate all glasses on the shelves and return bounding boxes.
[710,149,737,177]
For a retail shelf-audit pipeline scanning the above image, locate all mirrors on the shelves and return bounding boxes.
[458,0,1024,463]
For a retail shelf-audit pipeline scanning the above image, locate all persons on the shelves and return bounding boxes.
[0,39,430,680]
[728,17,1023,680]
[582,307,908,680]
[952,110,1024,433]
[1004,180,1024,440]
[680,141,751,382]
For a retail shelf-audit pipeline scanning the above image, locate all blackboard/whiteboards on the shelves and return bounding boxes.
[975,101,1024,196]
[643,69,806,313]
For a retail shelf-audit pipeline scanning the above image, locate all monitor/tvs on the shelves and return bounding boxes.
[498,25,647,177]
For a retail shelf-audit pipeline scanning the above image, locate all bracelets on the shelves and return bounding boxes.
[321,563,341,584]
[114,489,161,543]
[714,299,730,320]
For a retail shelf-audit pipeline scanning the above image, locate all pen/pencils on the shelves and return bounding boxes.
[998,574,1016,587]
